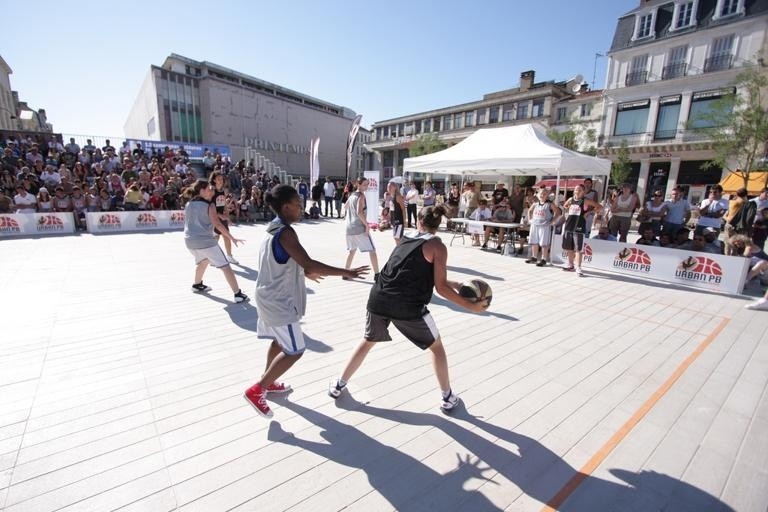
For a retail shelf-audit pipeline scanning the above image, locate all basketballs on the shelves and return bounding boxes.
[458,278,493,312]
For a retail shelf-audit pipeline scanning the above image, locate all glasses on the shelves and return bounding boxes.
[709,191,717,194]
[499,205,506,207]
[654,194,662,197]
[479,203,485,205]
[599,232,605,234]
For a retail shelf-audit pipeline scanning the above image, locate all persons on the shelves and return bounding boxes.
[13,186,37,212]
[637,191,668,238]
[752,208,768,249]
[517,196,535,255]
[342,181,354,203]
[186,179,250,304]
[71,185,88,231]
[372,208,391,230]
[343,177,381,283]
[456,181,471,233]
[470,200,491,246]
[0,189,13,213]
[53,187,71,212]
[295,177,308,220]
[148,190,169,211]
[165,189,181,210]
[421,182,436,211]
[139,187,150,210]
[209,172,240,264]
[447,187,460,232]
[309,202,320,220]
[407,182,418,227]
[636,223,657,247]
[528,189,559,266]
[202,146,257,172]
[227,186,270,226]
[36,187,54,213]
[728,189,756,236]
[335,180,343,219]
[695,185,728,239]
[124,185,140,211]
[244,185,370,419]
[726,235,767,288]
[312,180,322,216]
[655,230,674,247]
[386,183,408,244]
[480,202,513,253]
[561,184,604,276]
[695,227,721,254]
[579,178,597,238]
[325,205,489,412]
[111,191,125,210]
[509,184,525,222]
[324,176,336,219]
[595,227,617,241]
[179,187,188,210]
[744,290,767,310]
[750,189,767,224]
[661,186,692,243]
[673,229,694,248]
[85,189,98,211]
[323,263,370,281]
[240,173,280,185]
[375,222,377,223]
[491,181,509,213]
[609,183,636,241]
[465,181,484,232]
[0,133,198,190]
[98,190,111,211]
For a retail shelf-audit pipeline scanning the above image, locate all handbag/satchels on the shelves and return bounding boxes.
[636,208,651,221]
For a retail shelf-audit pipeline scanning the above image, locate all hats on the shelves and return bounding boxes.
[39,187,47,193]
[496,180,505,185]
[450,182,457,186]
[495,202,509,206]
[702,227,717,235]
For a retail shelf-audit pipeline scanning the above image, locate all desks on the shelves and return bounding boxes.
[449,218,522,255]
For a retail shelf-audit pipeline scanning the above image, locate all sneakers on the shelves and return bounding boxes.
[243,384,275,419]
[323,214,327,217]
[328,378,348,399]
[342,215,344,218]
[472,241,480,246]
[225,254,238,264]
[745,297,768,310]
[517,247,524,256]
[330,213,334,218]
[480,243,489,250]
[562,265,574,271]
[575,268,584,277]
[496,246,501,253]
[263,383,292,393]
[335,216,339,219]
[233,289,250,304]
[439,390,459,410]
[192,282,212,291]
[536,259,546,266]
[525,256,537,263]
